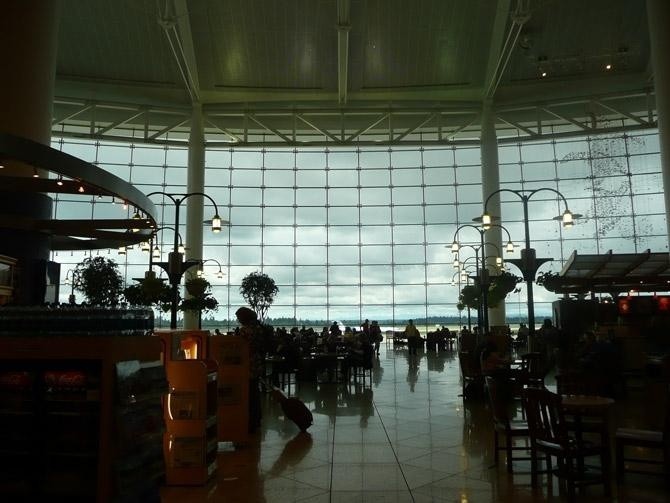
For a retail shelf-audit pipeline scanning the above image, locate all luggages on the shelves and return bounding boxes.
[274,387,313,430]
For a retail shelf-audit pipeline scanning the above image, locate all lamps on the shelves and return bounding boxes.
[444,187,584,331]
[117,192,230,328]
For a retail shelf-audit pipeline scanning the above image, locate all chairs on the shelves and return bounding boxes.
[471,352,668,499]
[262,328,458,398]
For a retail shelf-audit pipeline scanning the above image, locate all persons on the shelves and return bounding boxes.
[405,318,419,355]
[436,316,557,398]
[233,320,383,383]
[236,307,271,432]
[408,357,420,392]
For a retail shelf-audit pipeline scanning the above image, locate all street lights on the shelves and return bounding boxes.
[116,192,226,330]
[446,186,583,408]
